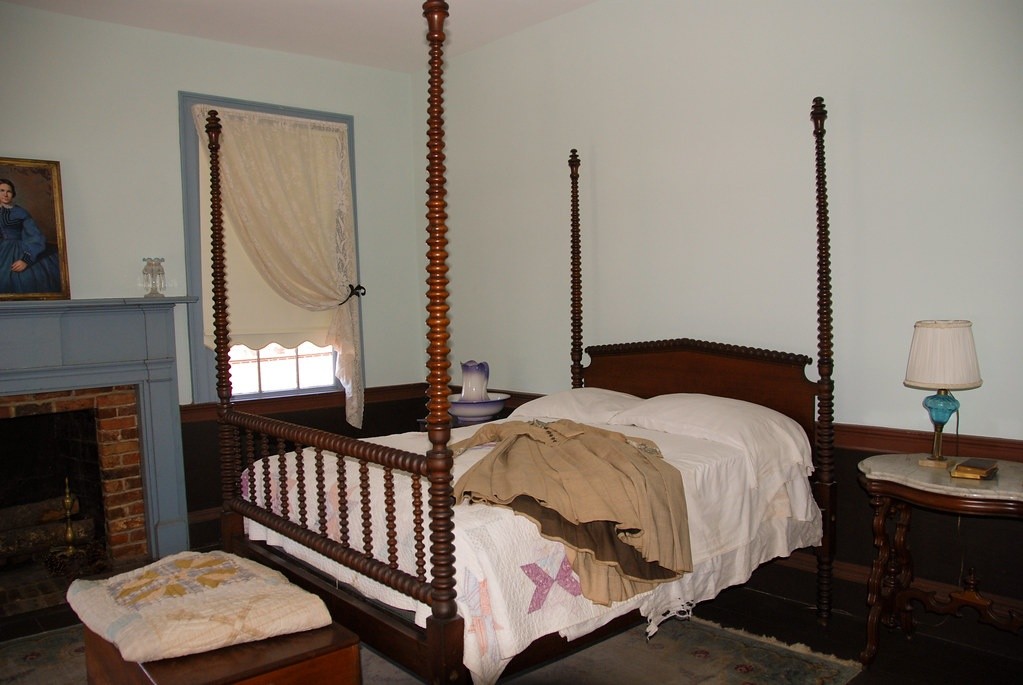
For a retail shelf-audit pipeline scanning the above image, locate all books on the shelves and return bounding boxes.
[950,457,1000,481]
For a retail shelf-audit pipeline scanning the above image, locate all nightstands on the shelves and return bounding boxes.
[416,407,515,431]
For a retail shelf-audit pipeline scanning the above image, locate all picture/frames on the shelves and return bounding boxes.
[0,156,72,300]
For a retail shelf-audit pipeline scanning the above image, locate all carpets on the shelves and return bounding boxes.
[361,609,862,685]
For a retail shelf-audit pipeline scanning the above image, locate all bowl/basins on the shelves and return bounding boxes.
[445,392,510,421]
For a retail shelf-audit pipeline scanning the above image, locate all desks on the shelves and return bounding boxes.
[83,622,362,685]
[857,450,1023,661]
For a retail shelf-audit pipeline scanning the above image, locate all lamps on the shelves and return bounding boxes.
[904,318,979,465]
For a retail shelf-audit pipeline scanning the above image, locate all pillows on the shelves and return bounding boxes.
[504,387,815,502]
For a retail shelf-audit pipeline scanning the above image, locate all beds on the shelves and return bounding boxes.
[214,338,835,685]
